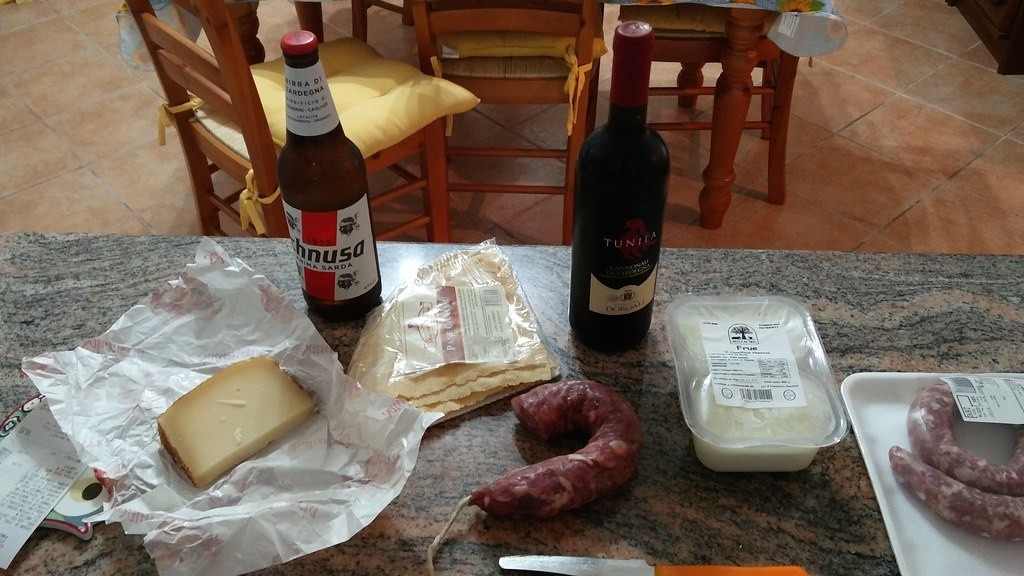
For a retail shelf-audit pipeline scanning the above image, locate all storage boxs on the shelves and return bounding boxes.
[664,294,849,473]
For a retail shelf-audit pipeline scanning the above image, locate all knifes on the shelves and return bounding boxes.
[499,554,809,576]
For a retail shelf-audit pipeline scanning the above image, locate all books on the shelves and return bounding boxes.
[0,393,114,541]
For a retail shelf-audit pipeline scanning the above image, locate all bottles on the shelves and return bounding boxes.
[276,31,382,322]
[570,22,670,355]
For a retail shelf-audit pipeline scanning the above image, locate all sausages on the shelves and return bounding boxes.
[466,380,642,518]
[888,381,1024,542]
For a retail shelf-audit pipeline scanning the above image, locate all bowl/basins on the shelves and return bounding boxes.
[665,296,848,471]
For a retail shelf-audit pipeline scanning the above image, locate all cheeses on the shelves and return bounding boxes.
[158,355,318,489]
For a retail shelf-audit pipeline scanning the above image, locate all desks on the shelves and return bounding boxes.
[0,232,1024,576]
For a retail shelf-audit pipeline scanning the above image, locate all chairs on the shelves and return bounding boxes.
[619,0,815,232]
[126,0,481,244]
[406,0,604,248]
